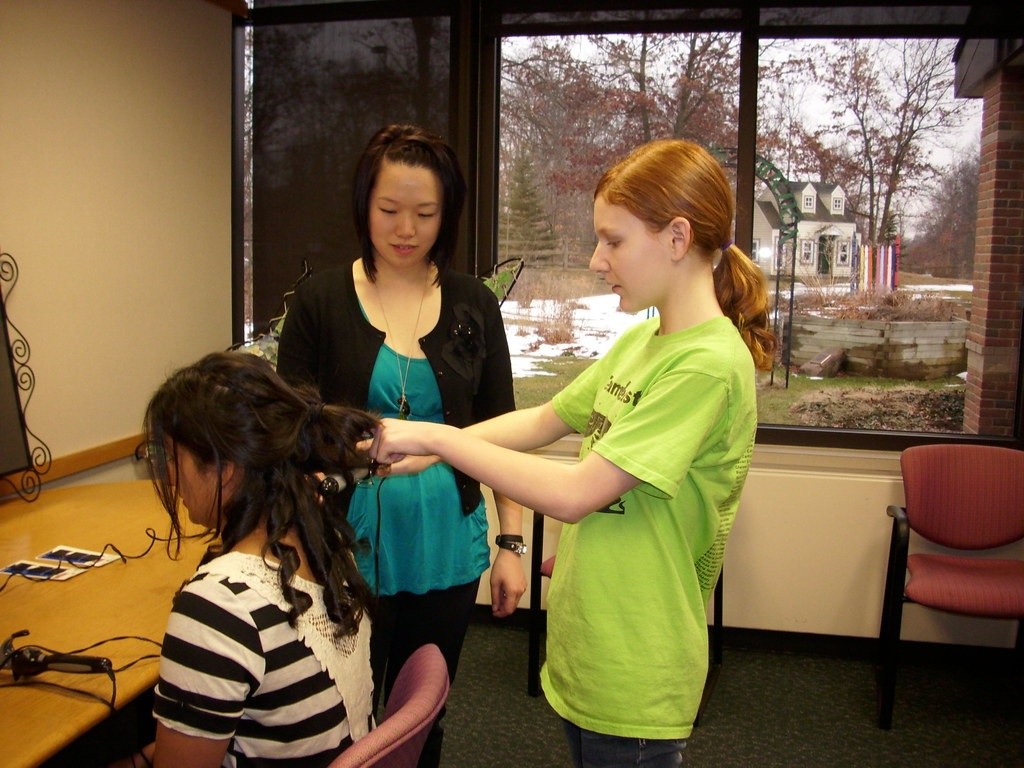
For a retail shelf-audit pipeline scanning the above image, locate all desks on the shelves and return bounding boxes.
[0,480,224,768]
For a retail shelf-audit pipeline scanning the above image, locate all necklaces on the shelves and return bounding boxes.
[373,264,429,418]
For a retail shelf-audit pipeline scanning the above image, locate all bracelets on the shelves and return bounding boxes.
[138,749,153,768]
[130,754,136,768]
[495,535,523,544]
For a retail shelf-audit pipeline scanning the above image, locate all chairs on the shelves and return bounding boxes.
[528,510,723,728]
[328,643,451,768]
[874,444,1024,730]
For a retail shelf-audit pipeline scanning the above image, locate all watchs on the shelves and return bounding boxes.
[499,542,527,554]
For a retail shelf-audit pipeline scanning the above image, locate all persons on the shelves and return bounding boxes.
[277,125,527,768]
[345,140,777,768]
[107,351,386,768]
